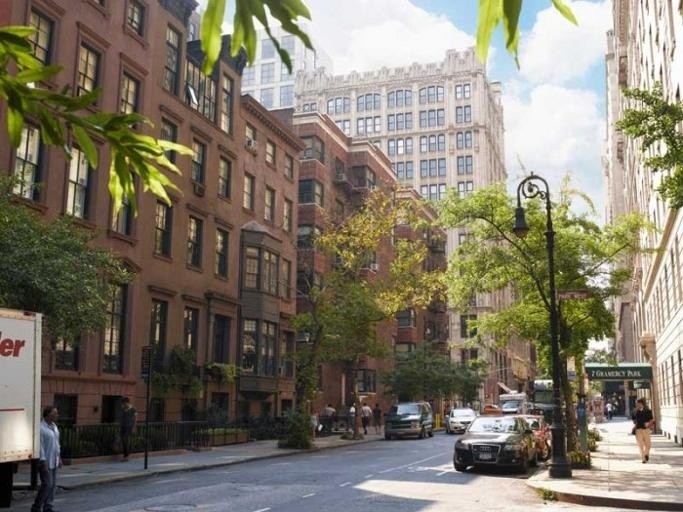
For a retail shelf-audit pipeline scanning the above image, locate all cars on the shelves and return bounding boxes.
[383,401,435,440]
[444,379,553,472]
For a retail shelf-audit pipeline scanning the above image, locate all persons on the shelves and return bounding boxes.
[606,401,613,421]
[309,408,320,442]
[30,405,64,512]
[324,400,383,435]
[443,398,458,434]
[119,396,138,458]
[631,395,654,463]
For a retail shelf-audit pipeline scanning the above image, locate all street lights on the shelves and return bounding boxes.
[145,345,154,470]
[513,172,572,477]
